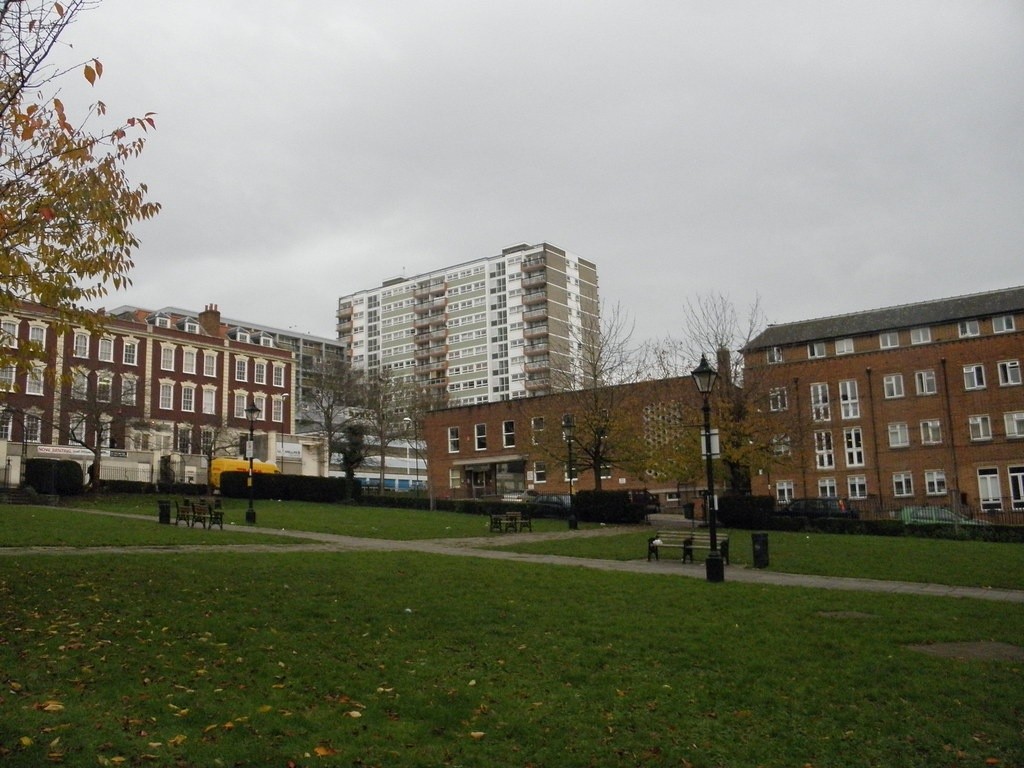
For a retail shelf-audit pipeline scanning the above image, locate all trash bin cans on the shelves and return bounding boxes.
[684,503,694,519]
[159,500,171,523]
[752,533,769,567]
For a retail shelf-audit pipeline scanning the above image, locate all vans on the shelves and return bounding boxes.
[776,495,860,519]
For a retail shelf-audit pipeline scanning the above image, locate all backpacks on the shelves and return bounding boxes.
[87,464,94,474]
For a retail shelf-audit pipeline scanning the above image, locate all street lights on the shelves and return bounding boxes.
[281,392,289,474]
[403,418,418,490]
[561,412,577,530]
[243,399,263,523]
[689,353,724,585]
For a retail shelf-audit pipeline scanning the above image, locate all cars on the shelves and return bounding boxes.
[628,489,660,513]
[533,493,575,509]
[900,506,993,526]
[500,489,541,503]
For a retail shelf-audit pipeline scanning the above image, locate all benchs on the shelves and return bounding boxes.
[175,496,224,530]
[646,529,729,564]
[490,513,533,532]
